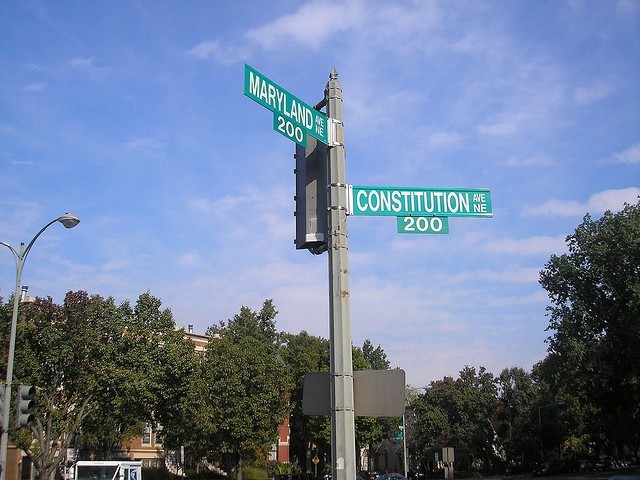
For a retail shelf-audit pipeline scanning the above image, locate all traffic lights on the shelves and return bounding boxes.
[17,384,36,429]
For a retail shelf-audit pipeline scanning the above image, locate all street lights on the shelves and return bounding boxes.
[0,211,81,480]
